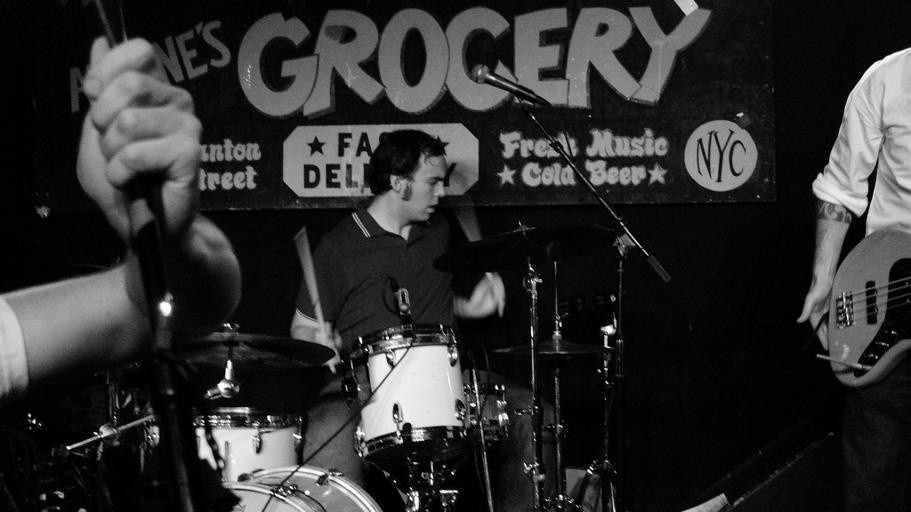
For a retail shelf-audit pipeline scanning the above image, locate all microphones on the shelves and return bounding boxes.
[391,277,412,325]
[470,62,546,109]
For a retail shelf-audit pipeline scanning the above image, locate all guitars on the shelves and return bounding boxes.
[827,223,911,386]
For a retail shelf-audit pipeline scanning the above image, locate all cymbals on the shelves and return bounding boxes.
[467,224,576,271]
[494,337,616,357]
[172,332,335,367]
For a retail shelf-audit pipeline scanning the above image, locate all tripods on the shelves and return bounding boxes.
[565,258,634,511]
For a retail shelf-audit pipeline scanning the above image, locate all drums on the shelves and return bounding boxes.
[222,465,385,512]
[339,321,469,470]
[415,380,509,465]
[300,396,364,488]
[191,407,302,482]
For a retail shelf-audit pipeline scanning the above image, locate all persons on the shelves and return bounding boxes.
[290,128,555,512]
[797,46,911,512]
[0,37,241,407]
